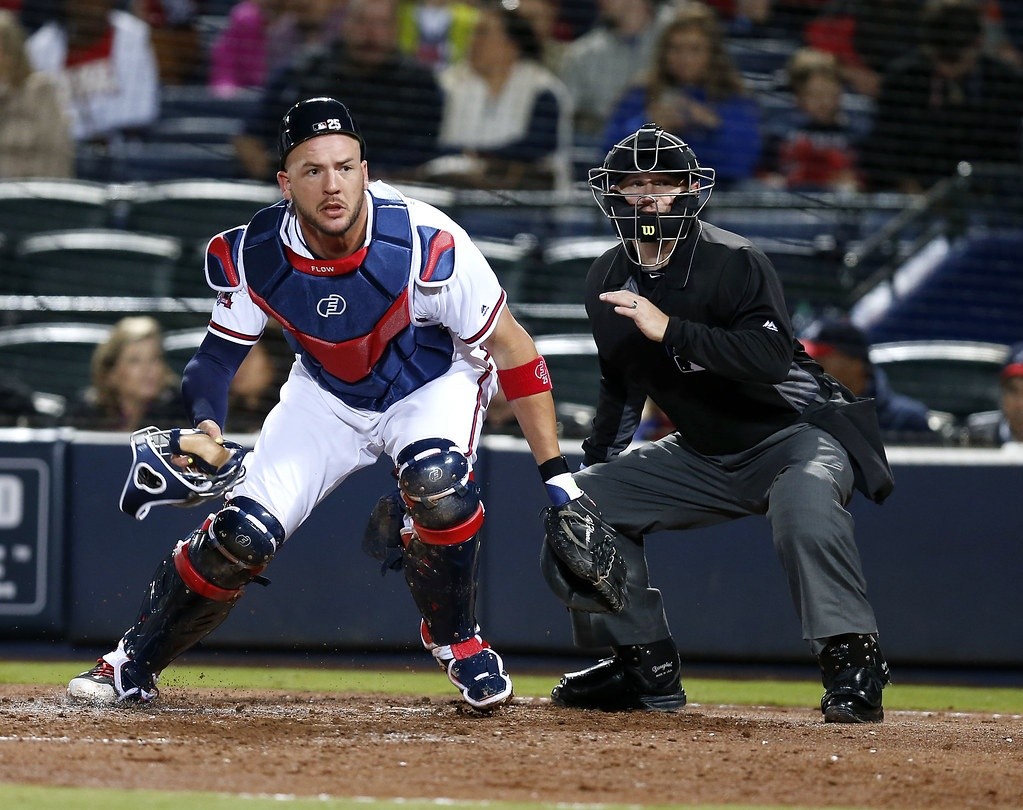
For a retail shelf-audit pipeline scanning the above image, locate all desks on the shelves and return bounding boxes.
[0,432,1023,689]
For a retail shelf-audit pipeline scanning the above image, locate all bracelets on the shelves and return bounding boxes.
[539,456,569,480]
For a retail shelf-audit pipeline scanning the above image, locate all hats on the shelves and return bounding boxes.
[799,321,870,364]
[1001,341,1023,378]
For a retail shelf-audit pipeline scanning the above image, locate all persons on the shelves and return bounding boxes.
[65,97,627,711]
[226,324,295,431]
[0,0,1023,195]
[793,311,1023,447]
[476,382,524,438]
[60,314,182,433]
[540,123,886,724]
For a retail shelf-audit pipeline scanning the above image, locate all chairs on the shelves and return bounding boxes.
[0,173,1023,416]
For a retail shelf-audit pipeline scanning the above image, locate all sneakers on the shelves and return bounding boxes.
[66,659,155,706]
[550,640,687,712]
[821,661,885,723]
[420,618,514,714]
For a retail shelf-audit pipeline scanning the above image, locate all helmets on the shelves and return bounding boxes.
[279,97,366,173]
[587,122,715,267]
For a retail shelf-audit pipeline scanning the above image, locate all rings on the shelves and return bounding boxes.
[632,301,637,308]
